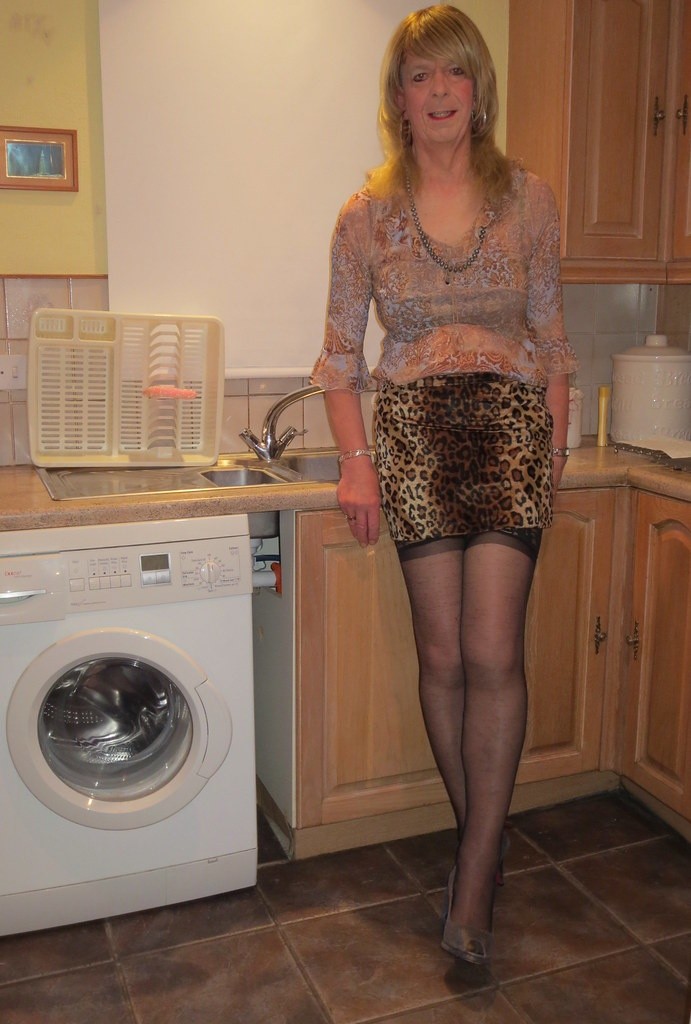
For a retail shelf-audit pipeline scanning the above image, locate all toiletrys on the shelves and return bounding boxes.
[596,385,612,446]
[566,387,585,449]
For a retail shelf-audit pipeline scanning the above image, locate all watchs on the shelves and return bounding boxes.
[552,446,570,458]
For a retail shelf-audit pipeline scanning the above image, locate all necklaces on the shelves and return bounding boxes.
[404,151,488,273]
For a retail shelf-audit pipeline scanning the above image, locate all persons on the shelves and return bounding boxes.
[317,6,575,970]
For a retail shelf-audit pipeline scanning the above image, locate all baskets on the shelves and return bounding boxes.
[28,308,224,467]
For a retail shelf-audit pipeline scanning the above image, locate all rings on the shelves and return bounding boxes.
[344,514,356,521]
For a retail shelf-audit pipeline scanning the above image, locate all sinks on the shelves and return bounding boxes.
[275,451,377,482]
[197,467,288,487]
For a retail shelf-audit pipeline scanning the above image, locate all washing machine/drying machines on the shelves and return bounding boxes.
[0,515,260,938]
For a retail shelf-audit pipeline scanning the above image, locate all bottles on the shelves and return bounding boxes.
[597,386,610,447]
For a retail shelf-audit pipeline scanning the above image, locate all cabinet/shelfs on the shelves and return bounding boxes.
[254,486,691,862]
[507,0,691,285]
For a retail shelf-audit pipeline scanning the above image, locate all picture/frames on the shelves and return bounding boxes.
[0,125,79,193]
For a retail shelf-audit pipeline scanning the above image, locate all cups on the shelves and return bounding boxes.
[566,387,583,448]
[610,333,691,447]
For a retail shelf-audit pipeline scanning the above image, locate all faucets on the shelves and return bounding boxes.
[260,383,326,461]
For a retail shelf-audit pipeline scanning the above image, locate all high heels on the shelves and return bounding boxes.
[442,864,499,963]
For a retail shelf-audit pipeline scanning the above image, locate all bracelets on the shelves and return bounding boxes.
[337,448,372,465]
